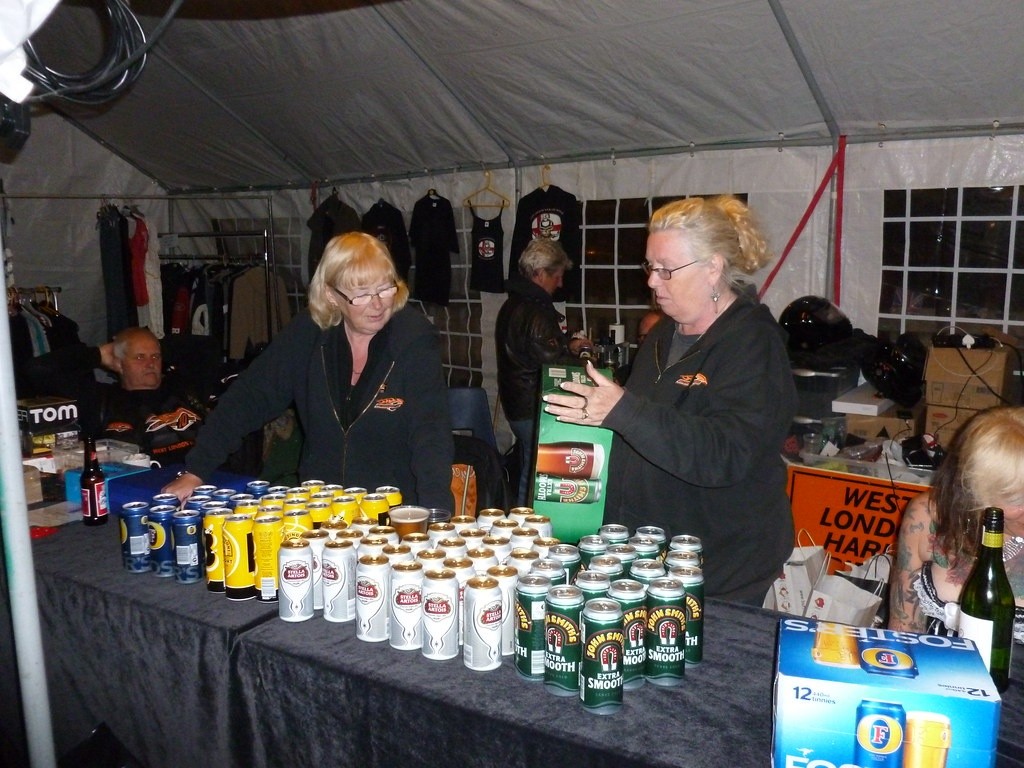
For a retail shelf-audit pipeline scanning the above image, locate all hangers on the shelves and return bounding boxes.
[541,165,549,186]
[92,194,148,232]
[427,175,444,198]
[462,170,510,209]
[7,285,63,327]
[373,183,388,208]
[175,253,264,285]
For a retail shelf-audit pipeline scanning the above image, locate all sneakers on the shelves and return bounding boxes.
[854,327,927,406]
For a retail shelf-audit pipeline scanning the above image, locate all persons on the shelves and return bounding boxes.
[159,232,456,525]
[888,407,1024,645]
[615,311,665,389]
[495,238,591,509]
[542,195,795,609]
[29,327,209,469]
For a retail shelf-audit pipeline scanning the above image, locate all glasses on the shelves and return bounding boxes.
[641,260,699,280]
[330,279,399,307]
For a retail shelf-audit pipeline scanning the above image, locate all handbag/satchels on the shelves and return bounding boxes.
[775,526,898,630]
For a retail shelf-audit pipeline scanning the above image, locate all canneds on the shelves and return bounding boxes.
[514,524,704,715]
[202,479,402,604]
[277,507,560,670]
[854,700,906,768]
[119,479,292,584]
[579,346,592,359]
[535,471,602,504]
[854,628,919,679]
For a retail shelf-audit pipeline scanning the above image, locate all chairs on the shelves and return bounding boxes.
[446,387,513,484]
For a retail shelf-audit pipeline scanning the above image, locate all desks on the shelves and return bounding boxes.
[28,500,1024,768]
[782,462,933,571]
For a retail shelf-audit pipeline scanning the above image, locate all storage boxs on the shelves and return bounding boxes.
[528,364,614,542]
[832,326,1021,451]
[16,395,259,514]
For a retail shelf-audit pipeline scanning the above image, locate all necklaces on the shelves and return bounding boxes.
[352,370,361,375]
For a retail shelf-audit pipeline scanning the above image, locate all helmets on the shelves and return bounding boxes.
[783,296,855,368]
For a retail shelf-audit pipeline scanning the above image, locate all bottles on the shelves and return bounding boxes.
[597,329,619,369]
[958,507,1016,695]
[79,435,109,527]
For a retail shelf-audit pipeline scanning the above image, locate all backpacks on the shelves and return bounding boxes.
[451,435,514,515]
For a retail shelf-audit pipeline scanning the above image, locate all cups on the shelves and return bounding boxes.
[388,504,431,542]
[902,713,955,768]
[535,441,605,481]
[427,508,452,530]
[811,623,859,670]
[801,434,822,466]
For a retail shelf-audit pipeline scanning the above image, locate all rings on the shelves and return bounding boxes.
[582,397,588,409]
[582,409,589,419]
